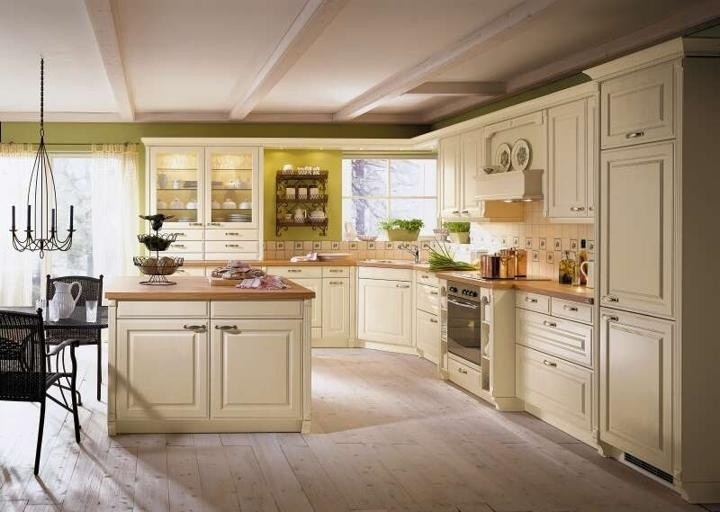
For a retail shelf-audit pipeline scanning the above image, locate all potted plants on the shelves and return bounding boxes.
[379,218,423,241]
[446,222,470,244]
[433,228,448,241]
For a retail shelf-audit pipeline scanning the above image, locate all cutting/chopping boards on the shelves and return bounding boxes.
[208,278,242,287]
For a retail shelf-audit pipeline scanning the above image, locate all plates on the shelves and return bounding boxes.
[495,138,530,173]
[183,181,223,188]
[228,213,250,222]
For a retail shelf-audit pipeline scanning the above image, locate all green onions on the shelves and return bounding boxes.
[423,235,480,272]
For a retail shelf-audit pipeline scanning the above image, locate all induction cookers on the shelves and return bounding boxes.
[445,272,552,302]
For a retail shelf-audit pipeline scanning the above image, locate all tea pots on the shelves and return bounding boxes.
[53,281,82,319]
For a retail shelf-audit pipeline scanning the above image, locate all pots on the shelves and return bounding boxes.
[479,247,528,279]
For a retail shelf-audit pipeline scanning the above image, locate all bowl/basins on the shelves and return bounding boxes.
[158,199,197,209]
[309,207,326,223]
[212,199,250,209]
[317,253,353,261]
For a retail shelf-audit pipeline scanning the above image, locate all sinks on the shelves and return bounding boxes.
[360,259,413,265]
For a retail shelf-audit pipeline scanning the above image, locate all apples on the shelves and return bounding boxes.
[141,257,157,276]
[156,258,175,274]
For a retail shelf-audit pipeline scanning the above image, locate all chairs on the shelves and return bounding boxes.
[0,273,108,476]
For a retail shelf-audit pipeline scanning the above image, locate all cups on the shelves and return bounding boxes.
[580,260,595,288]
[36,299,60,322]
[297,167,321,176]
[284,214,293,222]
[85,300,98,323]
[294,209,307,224]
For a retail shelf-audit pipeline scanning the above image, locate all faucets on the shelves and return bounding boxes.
[398,243,420,265]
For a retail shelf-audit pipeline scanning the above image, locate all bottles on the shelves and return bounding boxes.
[159,173,181,190]
[557,259,577,284]
[578,239,588,286]
[283,164,294,175]
[286,185,319,200]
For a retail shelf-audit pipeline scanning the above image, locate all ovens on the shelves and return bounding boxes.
[447,294,481,367]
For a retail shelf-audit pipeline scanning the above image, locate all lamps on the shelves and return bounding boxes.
[10,58,75,259]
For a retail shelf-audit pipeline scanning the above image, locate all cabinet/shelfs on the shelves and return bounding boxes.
[599,306,720,504]
[515,289,595,449]
[276,169,328,236]
[140,137,264,263]
[267,266,321,348]
[322,267,439,364]
[583,37,720,151]
[542,95,595,224]
[438,127,525,222]
[116,301,304,434]
[599,141,720,321]
